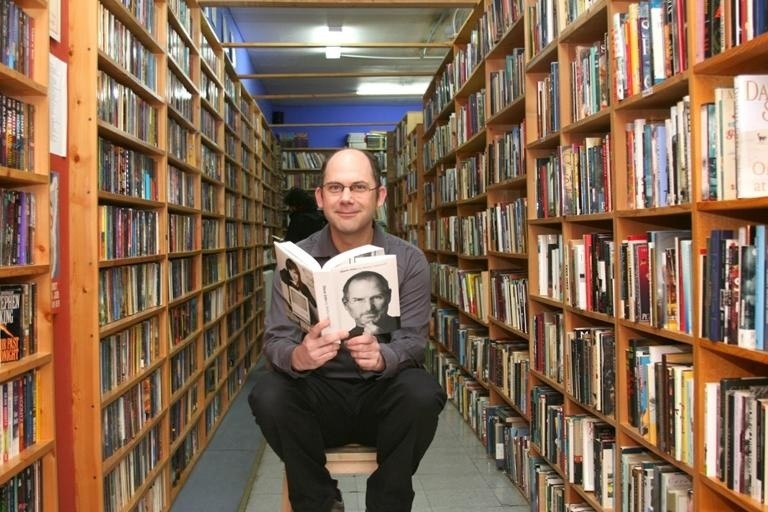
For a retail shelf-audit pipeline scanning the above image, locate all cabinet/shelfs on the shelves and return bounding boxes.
[284,146,386,231]
[0,0,284,512]
[386,0,768,511]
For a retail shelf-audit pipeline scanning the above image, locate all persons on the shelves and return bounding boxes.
[278,258,319,325]
[281,187,327,241]
[247,144,450,511]
[342,270,401,337]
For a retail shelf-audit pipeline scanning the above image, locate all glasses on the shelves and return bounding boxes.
[321,181,381,193]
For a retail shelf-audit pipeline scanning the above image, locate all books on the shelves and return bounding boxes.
[98,0,164,512]
[392,1,531,490]
[528,1,768,512]
[169,1,274,486]
[1,1,44,512]
[269,239,403,338]
[280,130,389,226]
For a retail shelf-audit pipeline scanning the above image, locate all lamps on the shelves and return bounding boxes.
[324,27,342,59]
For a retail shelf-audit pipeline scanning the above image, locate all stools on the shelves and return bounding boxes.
[283,442,380,512]
